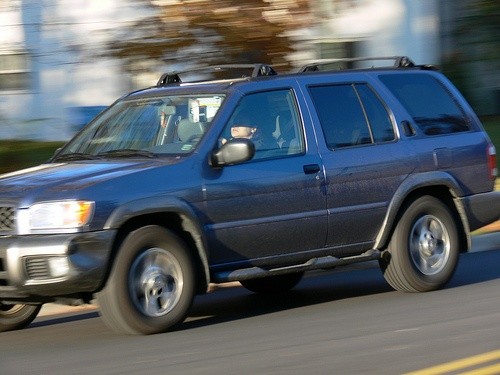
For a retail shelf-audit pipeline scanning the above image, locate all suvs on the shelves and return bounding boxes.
[0,52,498,338]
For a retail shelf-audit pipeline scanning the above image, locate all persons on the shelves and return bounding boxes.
[221,108,280,161]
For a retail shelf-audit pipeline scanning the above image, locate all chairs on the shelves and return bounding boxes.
[177,117,206,145]
[268,112,300,156]
[351,97,389,144]
